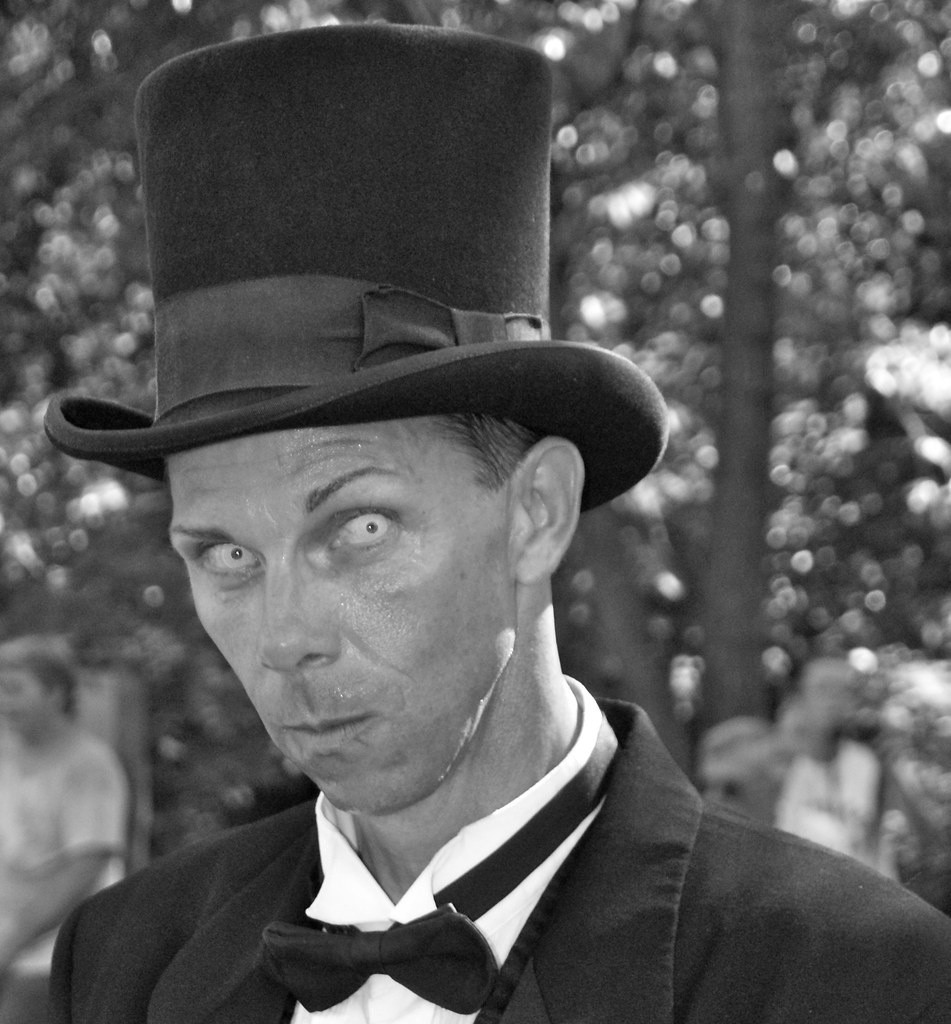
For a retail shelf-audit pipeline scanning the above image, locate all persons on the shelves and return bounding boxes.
[698,656,910,885]
[50,22,951,1024]
[0,630,128,1024]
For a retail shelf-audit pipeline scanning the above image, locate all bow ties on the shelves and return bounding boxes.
[262,711,620,1017]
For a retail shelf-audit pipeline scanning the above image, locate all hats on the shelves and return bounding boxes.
[42,23,669,515]
